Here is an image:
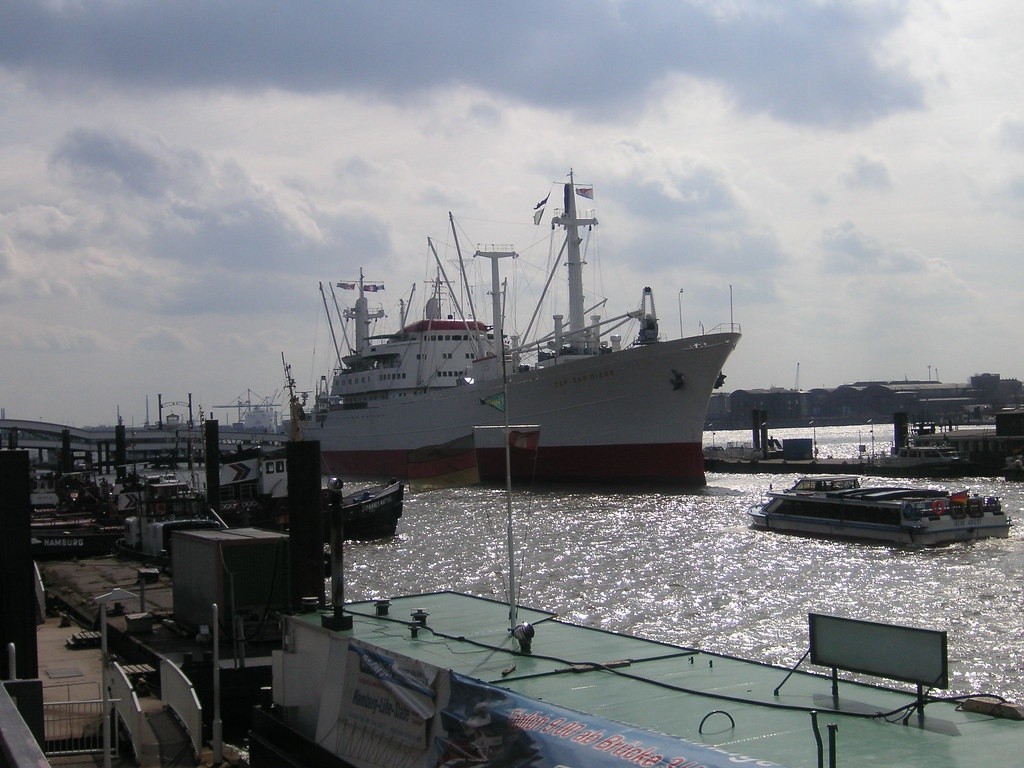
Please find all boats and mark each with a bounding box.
[276,165,743,487]
[751,473,1013,545]
[323,478,415,541]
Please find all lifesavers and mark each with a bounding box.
[904,503,914,517]
[966,498,986,519]
[948,500,967,520]
[932,500,945,516]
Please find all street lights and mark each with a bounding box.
[866,419,874,463]
[761,422,770,461]
[707,422,716,449]
[809,420,819,459]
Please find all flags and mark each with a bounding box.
[576,188,593,199]
[535,197,547,209]
[364,285,384,292]
[951,490,967,503]
[346,284,355,290]
[337,283,347,289]
[533,210,544,225]
